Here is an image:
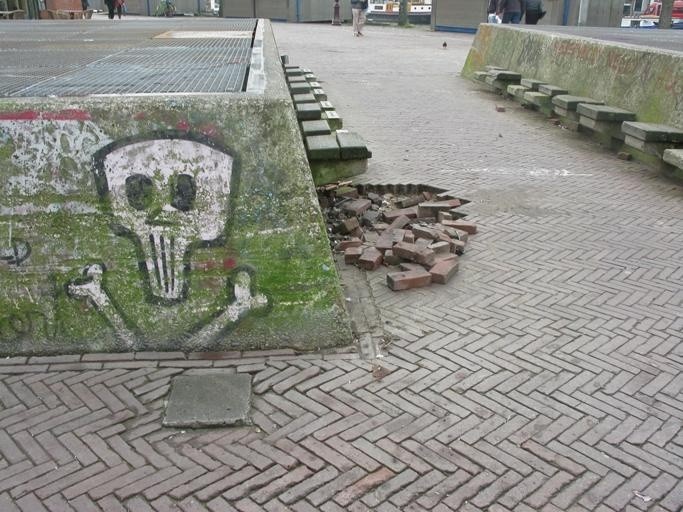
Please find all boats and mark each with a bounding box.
[364,0,432,25]
[619,4,683,29]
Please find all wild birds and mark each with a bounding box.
[443,41,447,48]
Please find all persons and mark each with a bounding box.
[104,0,114,19]
[486,0,499,24]
[522,0,547,25]
[495,0,523,25]
[349,0,369,38]
[112,0,124,20]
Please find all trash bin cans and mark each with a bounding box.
[332,0,341,26]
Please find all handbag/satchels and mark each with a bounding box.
[538,0,546,18]
